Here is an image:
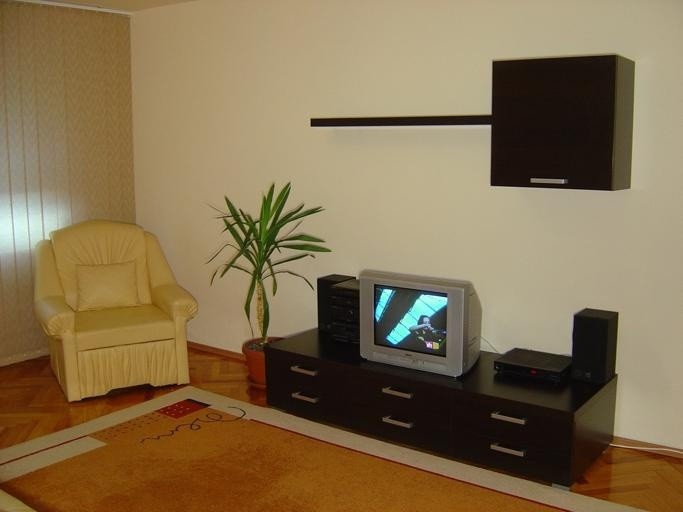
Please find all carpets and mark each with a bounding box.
[0,385,645,512]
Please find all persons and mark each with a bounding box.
[408,314,446,354]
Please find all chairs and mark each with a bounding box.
[33,220,200,403]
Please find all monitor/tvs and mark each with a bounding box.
[360,269,481,378]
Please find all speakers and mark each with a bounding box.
[574,308,618,381]
[317,275,354,336]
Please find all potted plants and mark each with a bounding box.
[201,181,332,385]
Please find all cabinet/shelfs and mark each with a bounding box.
[491,54,636,191]
[265,327,618,487]
